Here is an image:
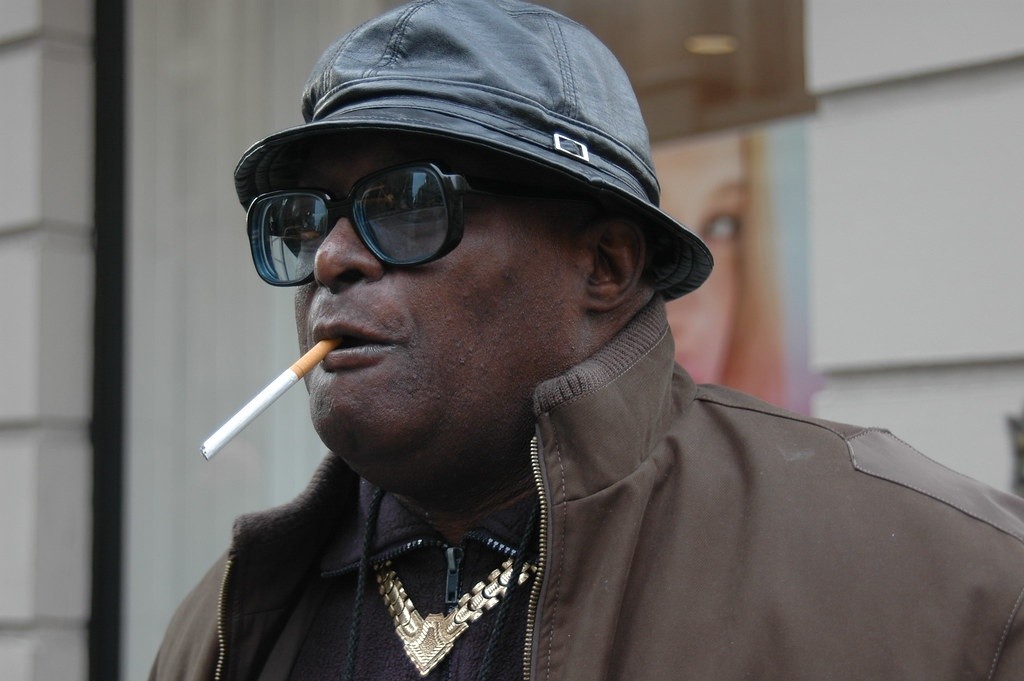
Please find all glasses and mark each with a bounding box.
[246,159,560,287]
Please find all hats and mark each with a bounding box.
[234,0,715,300]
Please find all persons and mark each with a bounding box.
[652,126,780,396]
[146,0,1024,680]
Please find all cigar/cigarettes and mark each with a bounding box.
[201,338,338,460]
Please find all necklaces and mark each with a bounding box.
[377,560,540,677]
